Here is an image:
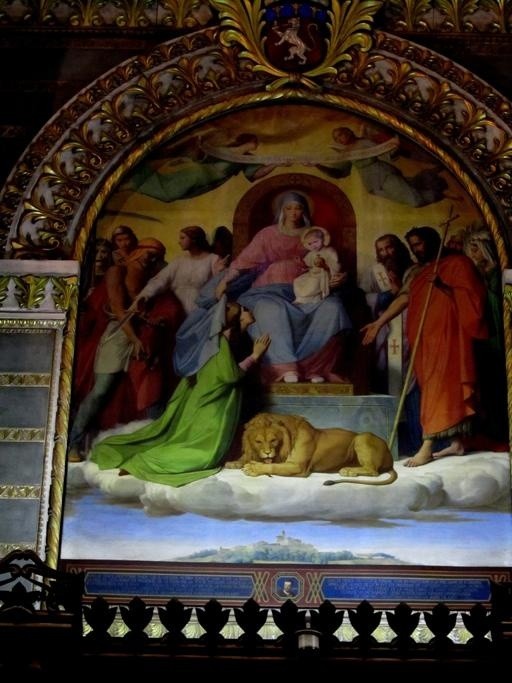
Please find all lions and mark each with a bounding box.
[223,411,397,487]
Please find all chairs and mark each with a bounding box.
[0,548,87,681]
[228,171,375,395]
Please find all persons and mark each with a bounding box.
[441,224,498,273]
[357,220,489,469]
[357,233,422,458]
[217,192,358,387]
[291,225,342,307]
